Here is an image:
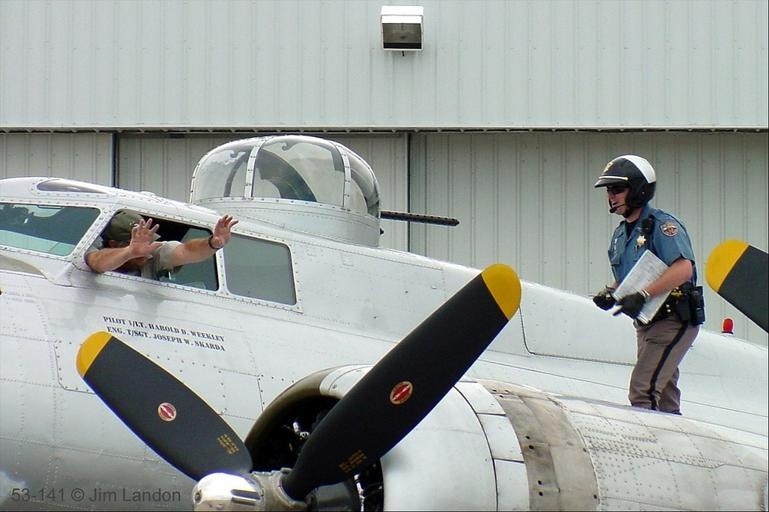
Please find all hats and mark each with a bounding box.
[101,210,160,245]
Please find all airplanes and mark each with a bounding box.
[0,133,769,512]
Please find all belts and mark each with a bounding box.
[633,300,673,329]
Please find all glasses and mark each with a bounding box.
[604,182,630,196]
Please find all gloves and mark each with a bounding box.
[611,291,651,319]
[593,286,617,311]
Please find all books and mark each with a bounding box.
[612,249,672,325]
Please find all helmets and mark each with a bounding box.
[594,153,656,213]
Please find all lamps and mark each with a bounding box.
[379,5,424,56]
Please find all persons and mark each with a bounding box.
[82,209,239,281]
[592,154,704,418]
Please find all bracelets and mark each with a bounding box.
[208,235,221,251]
[641,289,652,303]
